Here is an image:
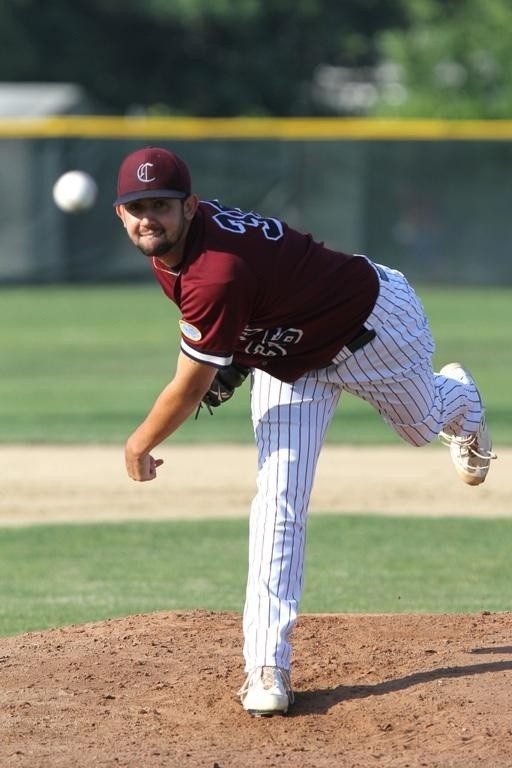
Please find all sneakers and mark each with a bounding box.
[438,361,498,484]
[236,666,295,716]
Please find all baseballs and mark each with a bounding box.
[50,169,98,213]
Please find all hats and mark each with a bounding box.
[112,146,191,207]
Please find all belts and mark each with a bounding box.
[321,262,390,368]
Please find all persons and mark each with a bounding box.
[104,142,503,717]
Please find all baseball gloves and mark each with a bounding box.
[194,360,250,420]
